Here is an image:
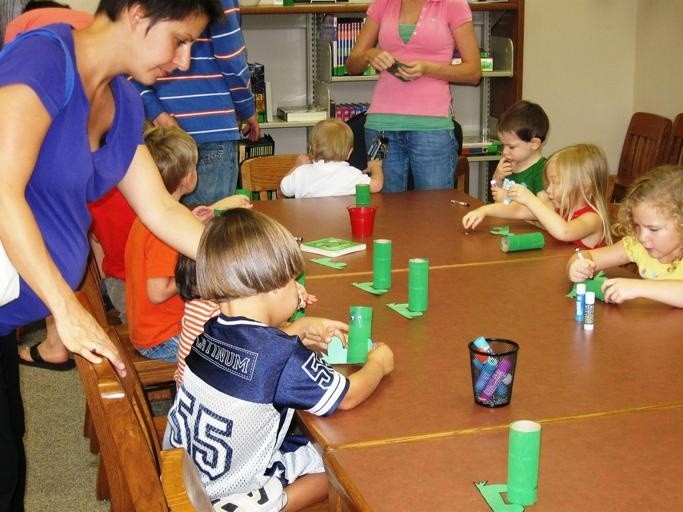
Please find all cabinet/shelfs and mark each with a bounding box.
[239,0,525,202]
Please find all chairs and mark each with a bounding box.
[602,111,674,209]
[239,154,313,199]
[67,257,197,512]
[660,113,682,168]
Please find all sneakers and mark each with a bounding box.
[211,476,287,512]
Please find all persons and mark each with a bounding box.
[175,209,395,511]
[0,0,318,511]
[88,125,138,320]
[174,250,326,395]
[121,1,258,206]
[462,142,611,252]
[487,101,550,206]
[566,166,683,310]
[345,1,482,194]
[277,118,384,200]
[124,124,254,361]
[4,1,94,372]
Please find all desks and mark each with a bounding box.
[323,403,683,512]
[179,187,585,279]
[289,254,683,450]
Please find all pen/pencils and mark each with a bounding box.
[451,199,471,207]
[576,249,595,282]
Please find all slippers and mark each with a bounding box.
[17,341,74,370]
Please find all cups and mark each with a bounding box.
[346,204,377,240]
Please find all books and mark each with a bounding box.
[276,102,326,121]
[324,15,377,76]
[329,100,371,121]
